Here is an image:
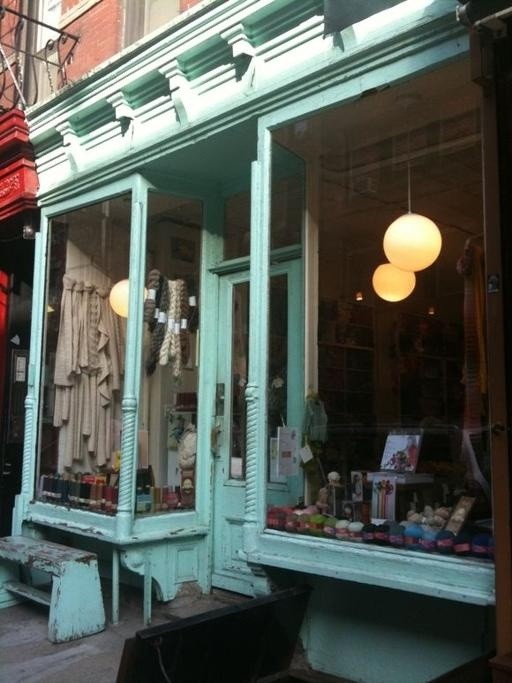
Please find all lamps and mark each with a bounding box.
[370,95,444,302]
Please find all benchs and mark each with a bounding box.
[0,533,109,646]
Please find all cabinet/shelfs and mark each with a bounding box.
[313,299,463,440]
